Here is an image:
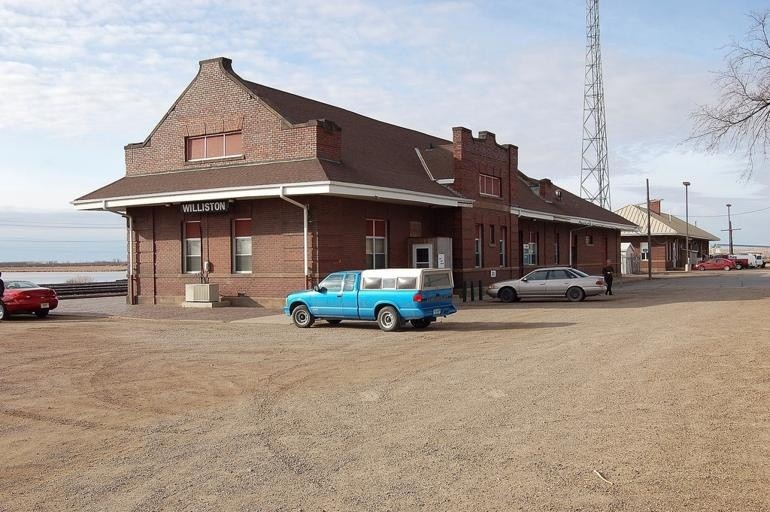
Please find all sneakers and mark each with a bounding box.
[605,292,614,296]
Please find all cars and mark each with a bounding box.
[1,280,59,323]
[485,265,608,303]
[694,252,767,271]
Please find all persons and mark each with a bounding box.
[601,258,616,296]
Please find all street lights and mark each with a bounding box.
[726,203,735,254]
[683,181,692,273]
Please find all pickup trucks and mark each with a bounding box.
[284,265,459,331]
